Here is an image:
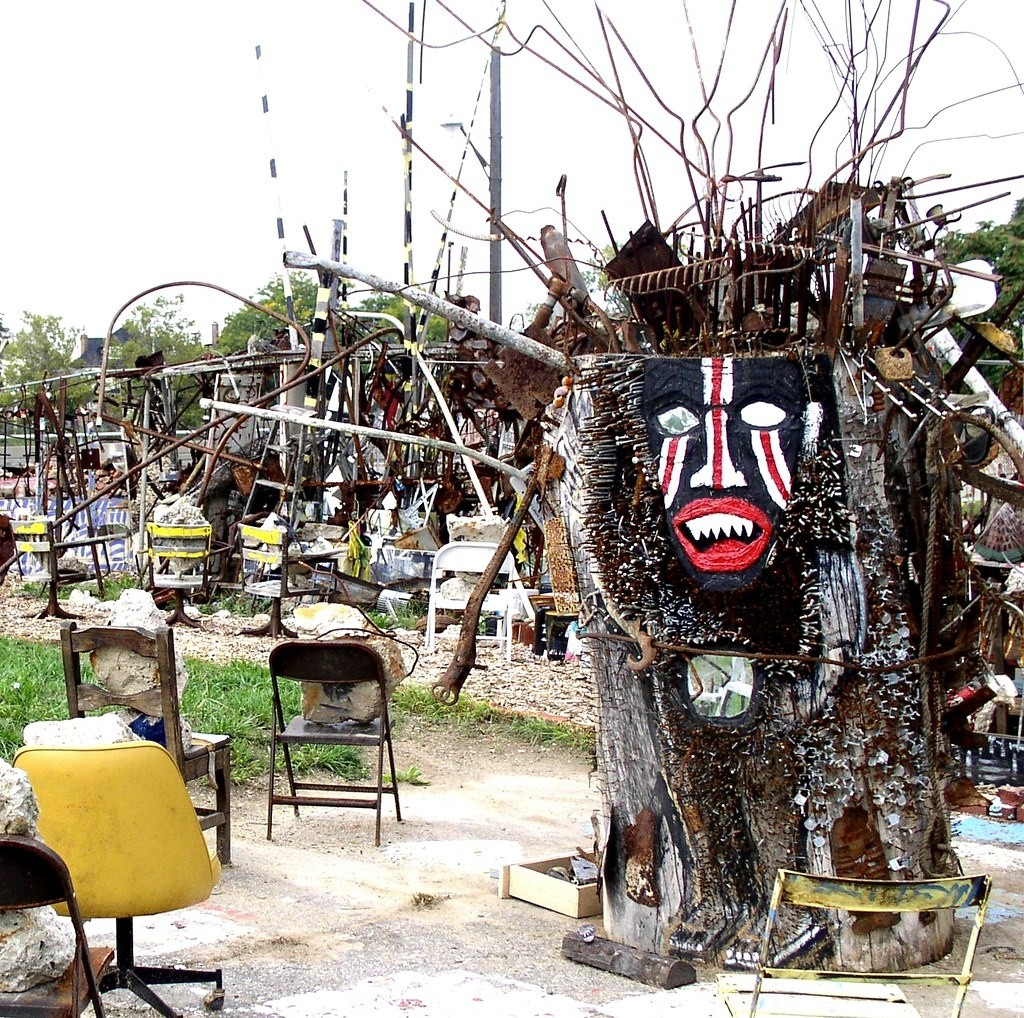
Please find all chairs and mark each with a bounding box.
[714,868,995,1017]
[0,833,114,1018]
[236,524,337,641]
[59,621,231,864]
[266,640,402,846]
[423,541,515,662]
[10,740,225,1018]
[145,523,233,629]
[8,520,128,621]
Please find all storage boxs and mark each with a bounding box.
[499,848,603,919]
